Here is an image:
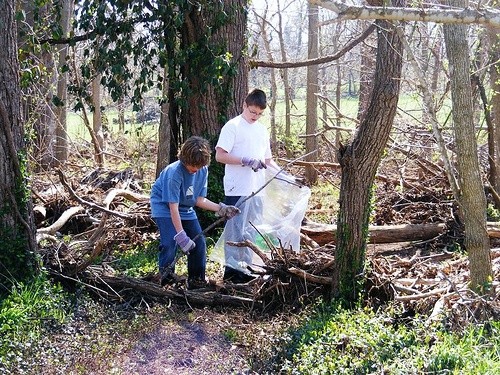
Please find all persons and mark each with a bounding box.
[215,89,309,284]
[150,136,242,290]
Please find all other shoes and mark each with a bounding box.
[187,280,210,289]
[161,276,182,288]
[222,266,261,283]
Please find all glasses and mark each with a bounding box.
[247,107,265,117]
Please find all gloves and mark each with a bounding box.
[215,202,242,220]
[174,230,196,255]
[295,176,311,187]
[241,156,267,173]
